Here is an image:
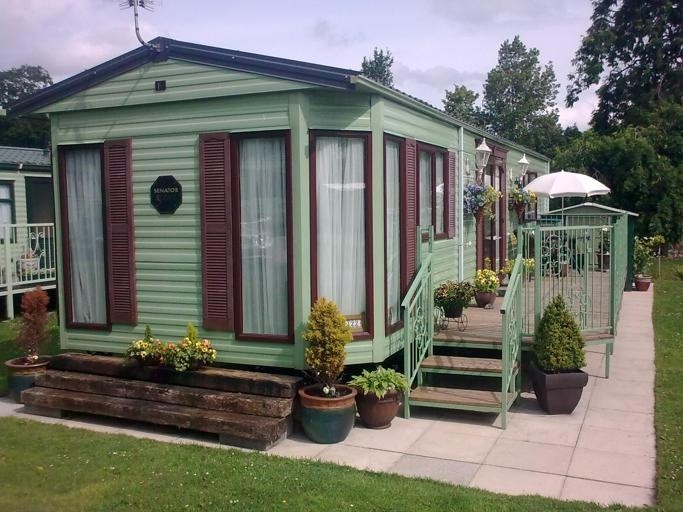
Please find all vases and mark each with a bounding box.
[476,292,494,307]
[434,303,468,333]
[515,204,526,221]
[474,205,482,225]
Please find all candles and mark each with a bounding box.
[295,297,358,444]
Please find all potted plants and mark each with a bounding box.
[629,238,654,291]
[593,218,611,268]
[3,286,51,404]
[347,365,408,430]
[527,293,588,414]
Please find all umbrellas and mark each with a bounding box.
[524,168,612,277]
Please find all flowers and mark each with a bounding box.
[510,187,536,205]
[500,256,534,276]
[434,276,476,306]
[464,181,500,213]
[125,324,218,372]
[473,268,498,292]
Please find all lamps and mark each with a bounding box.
[509,152,528,183]
[464,137,492,177]
